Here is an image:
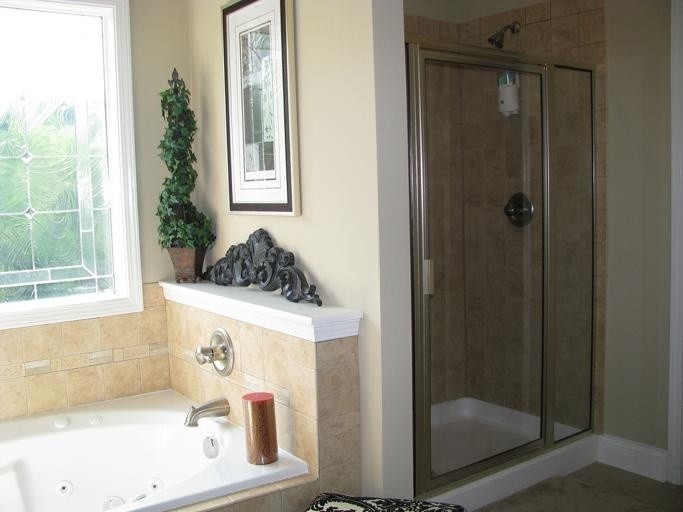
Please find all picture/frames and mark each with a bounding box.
[218,0,303,218]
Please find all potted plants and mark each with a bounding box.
[157,66,215,284]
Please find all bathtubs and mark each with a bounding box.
[0,387,308,512]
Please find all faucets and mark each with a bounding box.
[183,400,231,427]
